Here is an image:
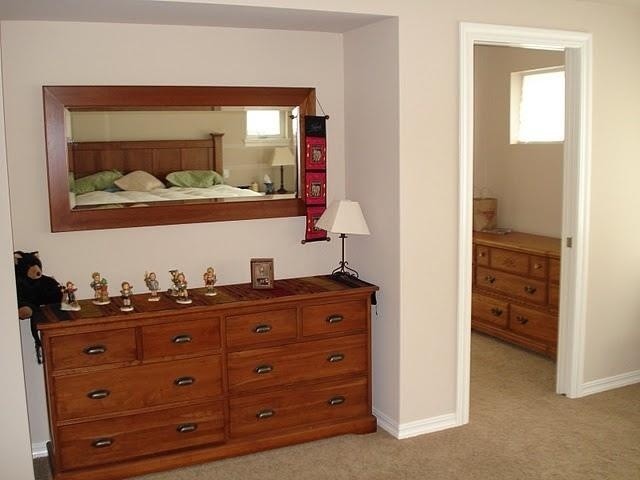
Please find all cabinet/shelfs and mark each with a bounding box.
[473,228,562,361]
[35,274,379,480]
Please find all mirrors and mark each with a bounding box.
[43,85,318,233]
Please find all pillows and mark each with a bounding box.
[69,169,224,197]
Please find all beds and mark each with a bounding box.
[67,133,263,207]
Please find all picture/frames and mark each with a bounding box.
[250,258,274,290]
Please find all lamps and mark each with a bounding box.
[314,199,371,281]
[268,146,296,193]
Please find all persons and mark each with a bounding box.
[149,273,159,297]
[64,282,77,304]
[205,267,216,294]
[120,281,133,308]
[176,273,188,301]
[169,270,179,291]
[90,271,102,301]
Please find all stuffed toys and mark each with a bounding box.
[15,251,63,363]
